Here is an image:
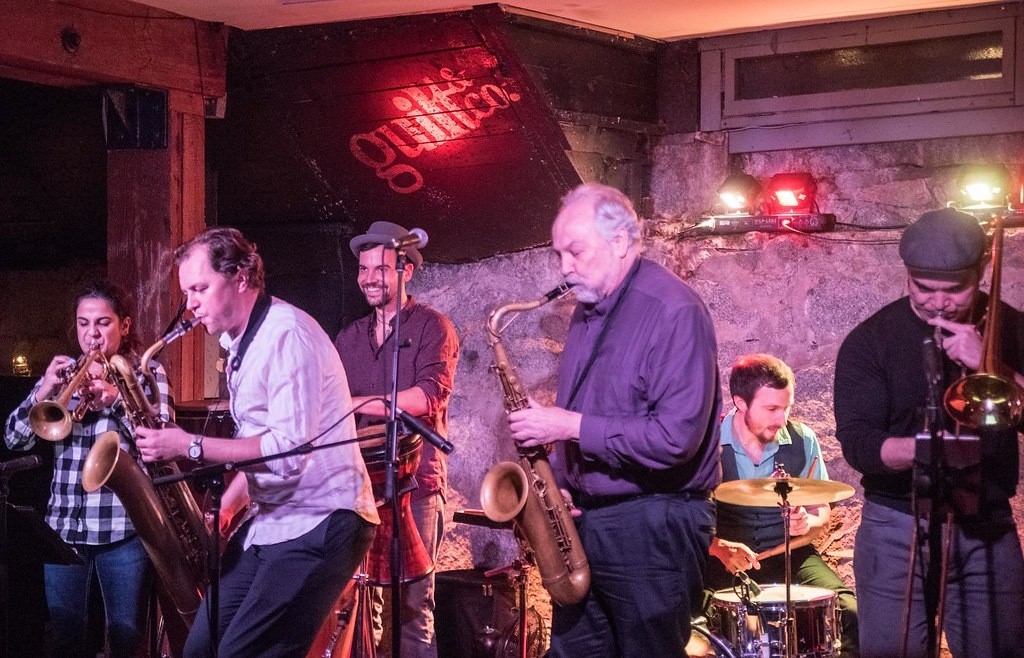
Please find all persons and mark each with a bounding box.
[503,184,722,658]
[329,223,459,657]
[834,209,1024,658]
[3,282,174,654]
[698,352,874,641]
[135,227,382,658]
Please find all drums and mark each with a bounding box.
[174,398,235,443]
[354,423,436,588]
[705,581,844,658]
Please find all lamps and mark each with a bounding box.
[954,161,1024,227]
[11,352,33,377]
[713,171,833,232]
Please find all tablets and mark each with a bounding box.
[911,435,982,515]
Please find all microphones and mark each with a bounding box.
[0,454,41,476]
[382,398,454,456]
[920,332,944,389]
[384,228,429,250]
[739,571,762,597]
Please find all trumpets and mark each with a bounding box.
[29,342,106,443]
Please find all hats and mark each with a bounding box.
[899,209,984,273]
[345,222,423,267]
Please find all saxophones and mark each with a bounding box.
[78,315,215,638]
[478,281,590,605]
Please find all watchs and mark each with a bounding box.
[187,434,206,464]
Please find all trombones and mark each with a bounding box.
[898,203,1024,658]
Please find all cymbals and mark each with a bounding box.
[711,474,856,510]
